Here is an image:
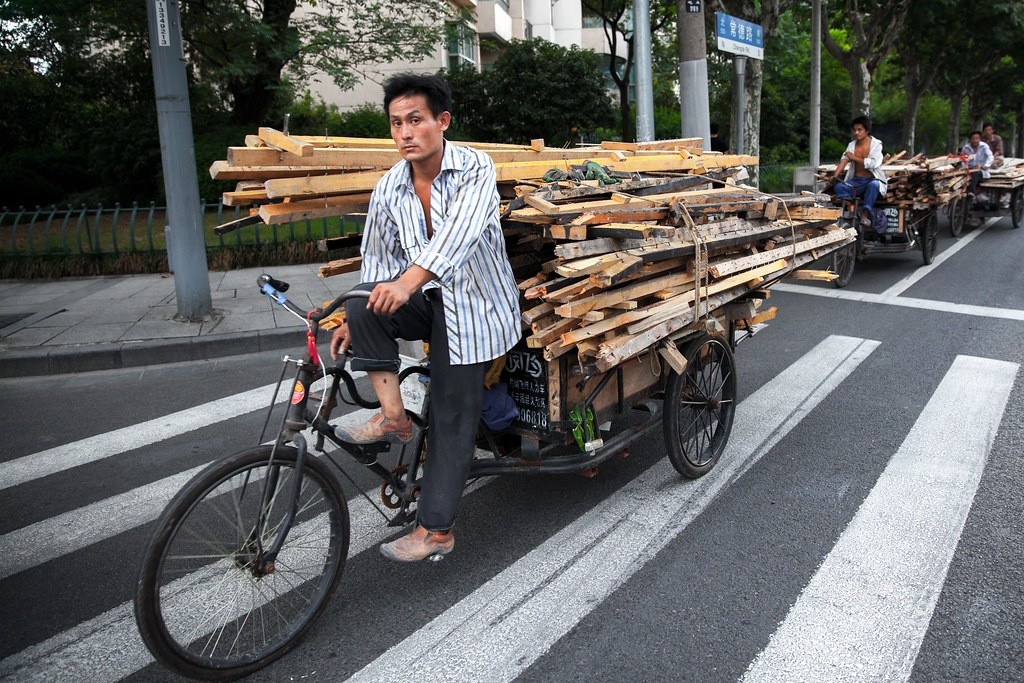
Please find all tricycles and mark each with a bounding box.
[947,168,1024,238]
[132,270,772,683]
[830,172,958,289]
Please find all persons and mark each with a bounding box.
[710,123,729,189]
[829,115,888,226]
[330,72,521,562]
[960,122,1004,198]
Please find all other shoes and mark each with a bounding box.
[966,192,974,204]
[860,215,871,226]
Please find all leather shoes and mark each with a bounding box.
[380,524,455,562]
[334,413,414,444]
